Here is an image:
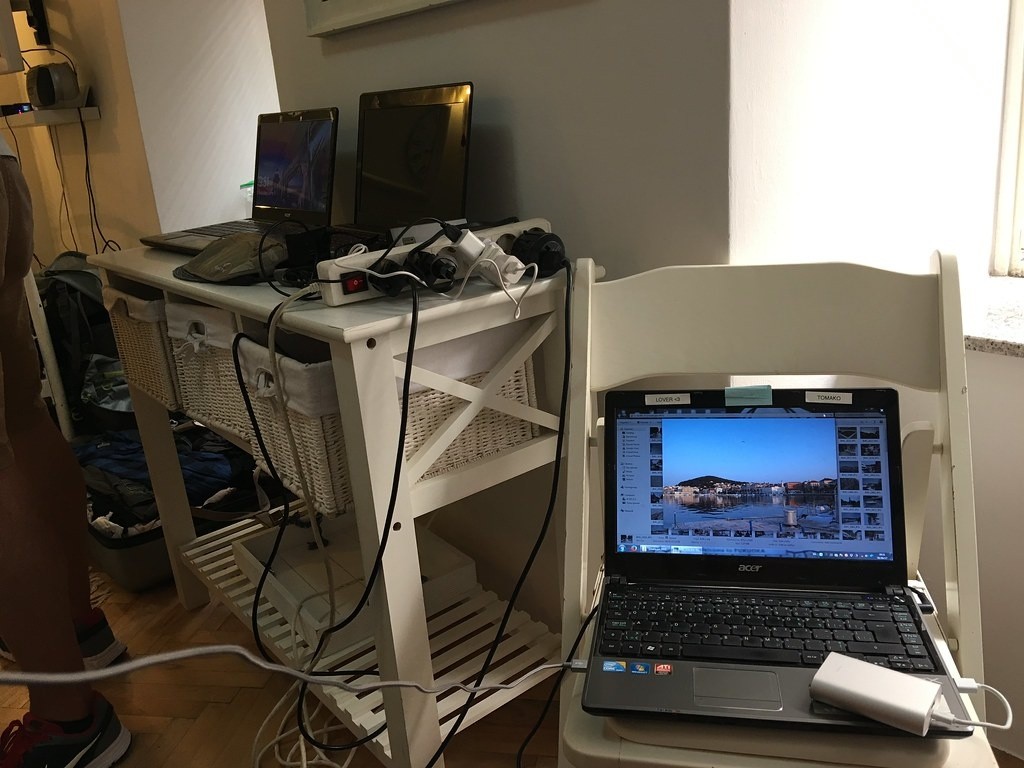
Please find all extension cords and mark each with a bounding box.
[317,217,550,307]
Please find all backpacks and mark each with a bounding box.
[31,251,138,437]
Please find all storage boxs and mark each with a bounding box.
[231,514,478,659]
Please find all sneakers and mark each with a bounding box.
[0,688,131,768]
[0,606,128,669]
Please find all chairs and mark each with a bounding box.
[557,249,999,768]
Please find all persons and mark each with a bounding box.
[0,139,132,768]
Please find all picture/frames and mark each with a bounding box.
[303,0,462,38]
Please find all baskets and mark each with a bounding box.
[101,285,183,412]
[232,334,541,520]
[164,303,250,442]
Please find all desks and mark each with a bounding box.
[86,245,607,768]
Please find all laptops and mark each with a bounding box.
[578,387,974,740]
[142,81,474,290]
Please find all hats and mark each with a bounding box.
[172,231,289,283]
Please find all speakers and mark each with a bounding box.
[37,61,85,110]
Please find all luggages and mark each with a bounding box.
[87,501,233,594]
